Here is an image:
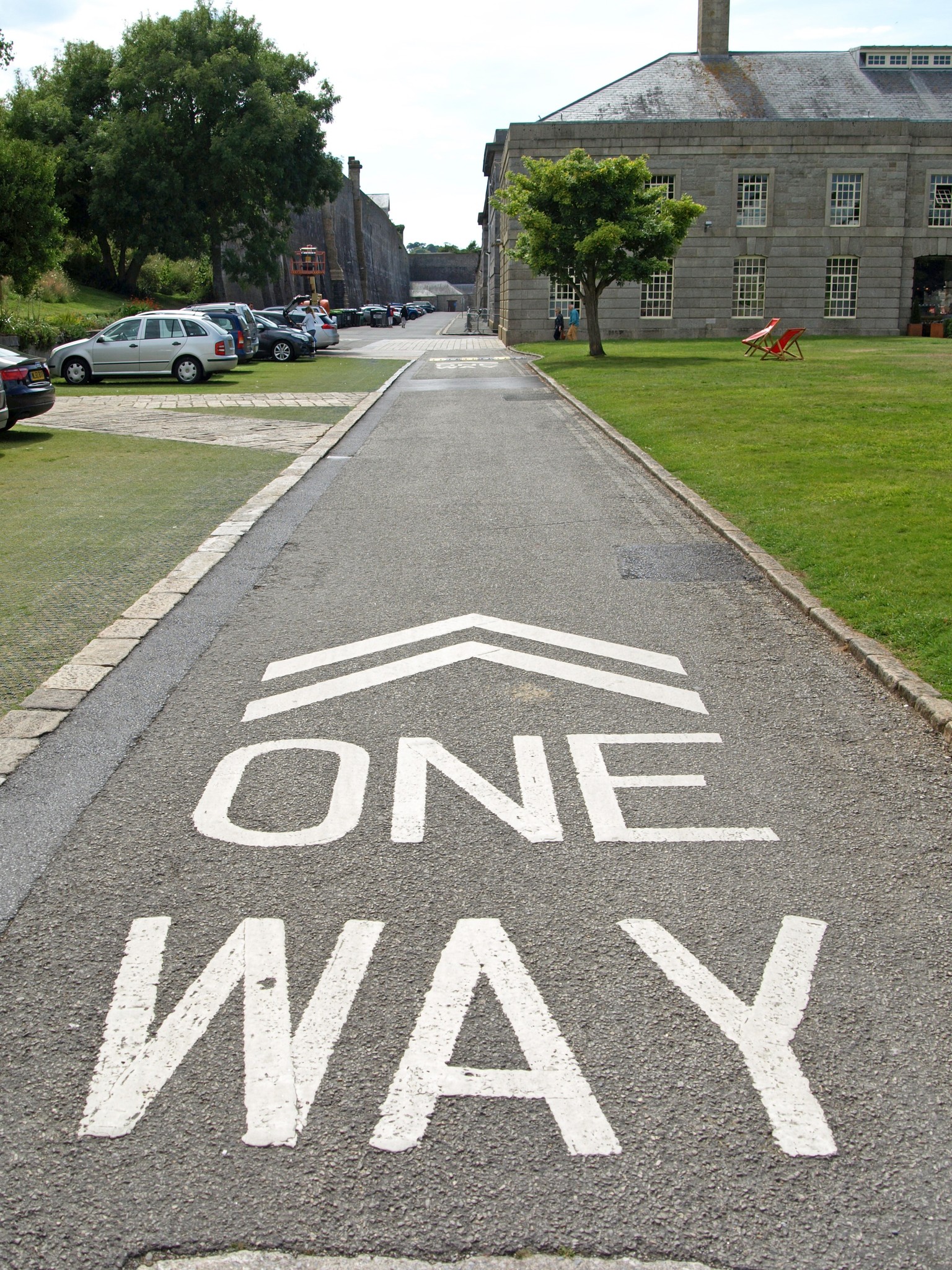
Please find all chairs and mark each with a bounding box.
[742,317,807,361]
[185,325,202,335]
[145,321,157,338]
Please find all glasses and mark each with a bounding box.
[250,306,254,307]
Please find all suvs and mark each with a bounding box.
[262,304,330,318]
[181,302,260,355]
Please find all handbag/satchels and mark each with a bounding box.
[390,308,394,316]
[562,329,566,339]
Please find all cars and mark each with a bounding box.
[190,309,254,364]
[0,344,57,432]
[218,314,317,362]
[271,310,339,349]
[358,306,402,326]
[251,294,313,333]
[103,310,213,352]
[48,314,238,385]
[362,303,434,321]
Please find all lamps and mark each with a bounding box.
[485,251,492,255]
[704,221,713,226]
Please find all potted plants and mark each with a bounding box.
[926,306,944,337]
[906,295,923,337]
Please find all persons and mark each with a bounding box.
[386,304,393,328]
[248,303,256,311]
[296,307,317,359]
[553,307,564,341]
[399,306,408,328]
[566,305,580,341]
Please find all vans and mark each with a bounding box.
[413,301,436,311]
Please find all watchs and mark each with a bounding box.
[559,327,560,328]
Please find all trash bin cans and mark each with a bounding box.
[356,312,364,326]
[370,308,382,327]
[382,309,388,327]
[329,308,357,329]
